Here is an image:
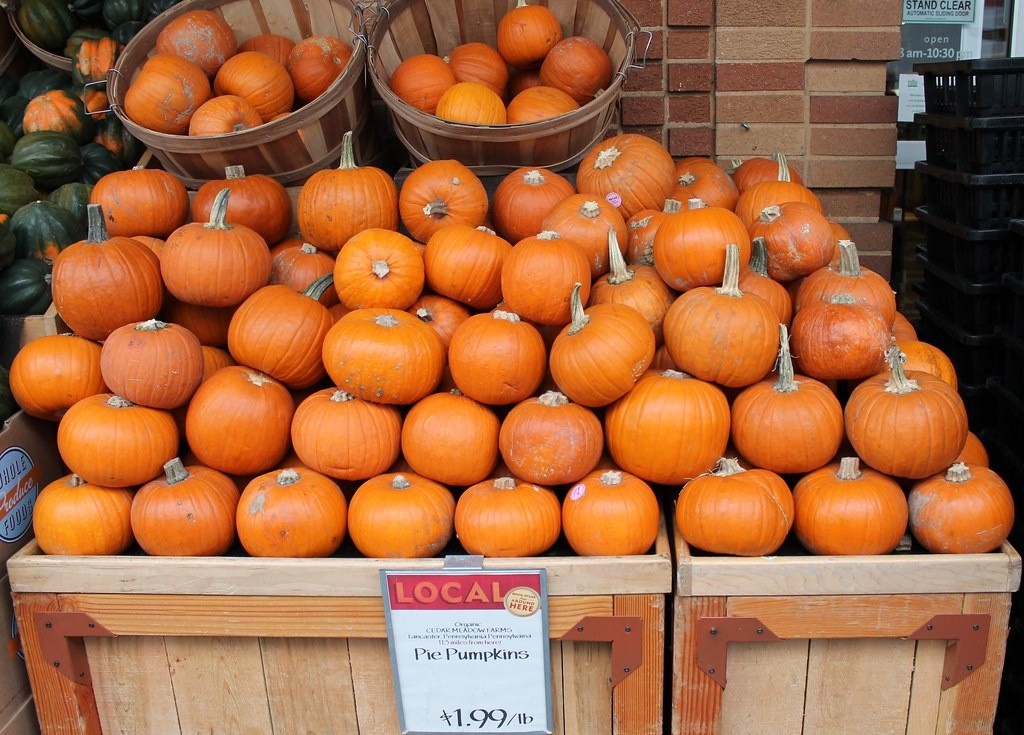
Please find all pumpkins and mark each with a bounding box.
[0,0,1015,557]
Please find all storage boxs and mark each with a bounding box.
[0,410,80,735]
[911,56,1024,735]
